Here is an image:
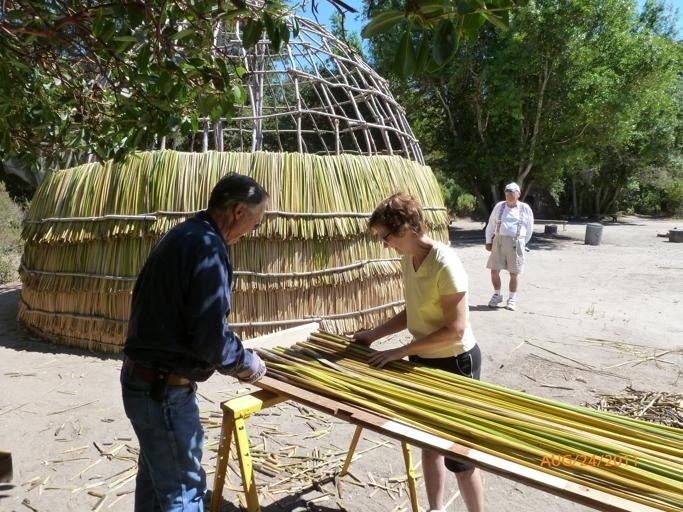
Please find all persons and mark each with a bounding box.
[352,192,487,512]
[121,170,270,512]
[484,182,534,311]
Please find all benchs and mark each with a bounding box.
[533,217,568,231]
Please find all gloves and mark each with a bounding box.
[236,349,267,384]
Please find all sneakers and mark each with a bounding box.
[488,294,503,308]
[506,299,516,311]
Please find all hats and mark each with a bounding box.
[504,182,520,192]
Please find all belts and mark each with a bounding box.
[125,358,190,385]
